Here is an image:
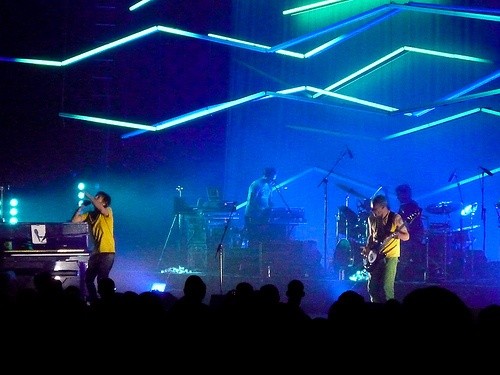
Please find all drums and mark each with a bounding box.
[332,211,472,283]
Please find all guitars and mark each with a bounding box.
[363,209,421,272]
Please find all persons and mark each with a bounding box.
[361,195,410,303]
[0,271,500,375]
[72,190,116,299]
[395,184,424,251]
[244,167,277,242]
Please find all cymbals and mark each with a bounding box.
[426,200,463,214]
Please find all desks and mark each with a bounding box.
[3,250,93,299]
[256,222,308,241]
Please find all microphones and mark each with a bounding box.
[479,165,493,176]
[347,146,354,158]
[224,202,237,205]
[448,172,456,183]
